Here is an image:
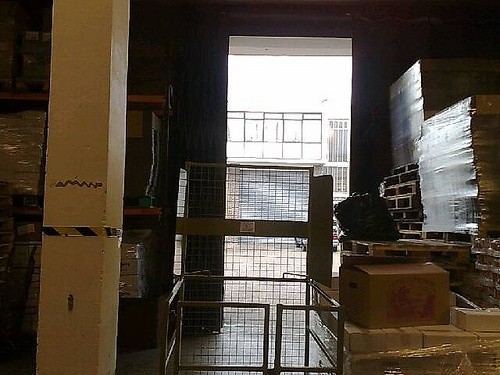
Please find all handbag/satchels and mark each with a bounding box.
[335,191,401,241]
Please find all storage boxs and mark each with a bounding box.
[308,271,500,375]
[338,255,449,328]
[4,220,42,339]
[119,229,158,299]
